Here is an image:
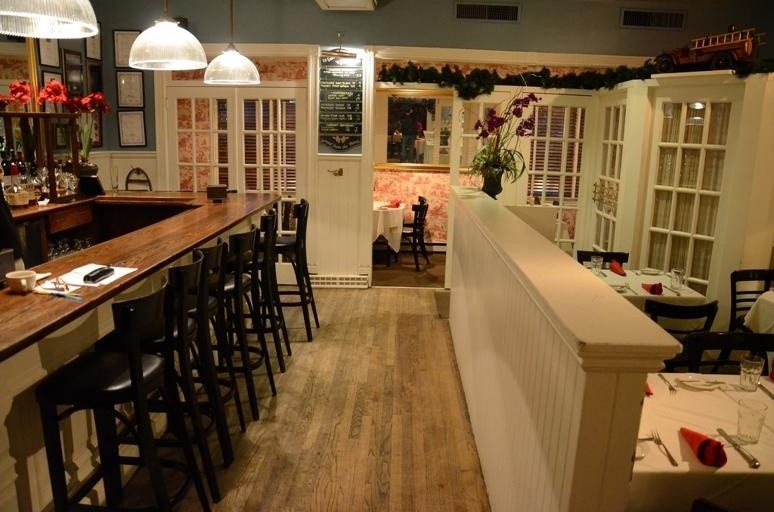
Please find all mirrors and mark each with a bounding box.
[372,87,456,174]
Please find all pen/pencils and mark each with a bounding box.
[32,289,83,302]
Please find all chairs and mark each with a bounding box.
[169,236,246,436]
[33,275,212,512]
[574,247,631,273]
[640,297,719,374]
[111,249,236,505]
[681,330,774,376]
[188,224,277,421]
[257,197,320,343]
[708,268,772,375]
[388,202,432,272]
[245,205,292,373]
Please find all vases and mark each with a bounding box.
[62,156,101,178]
[478,162,503,201]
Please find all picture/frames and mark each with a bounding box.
[35,35,62,69]
[112,28,142,70]
[116,110,148,149]
[60,47,87,97]
[37,77,67,116]
[115,71,145,110]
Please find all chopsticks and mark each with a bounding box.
[663,285,680,296]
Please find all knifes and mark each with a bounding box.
[716,428,761,469]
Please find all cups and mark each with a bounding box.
[739,354,766,393]
[591,256,603,277]
[671,267,684,290]
[5,270,37,293]
[737,398,768,443]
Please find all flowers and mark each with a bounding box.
[0,79,35,113]
[464,83,546,185]
[61,89,111,164]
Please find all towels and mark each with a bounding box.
[610,259,628,276]
[680,426,728,469]
[385,199,404,209]
[642,282,663,295]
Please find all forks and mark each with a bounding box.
[650,430,679,467]
[625,283,639,296]
[658,373,677,394]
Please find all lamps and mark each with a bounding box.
[203,0,265,89]
[0,0,100,42]
[127,0,206,76]
[319,29,359,61]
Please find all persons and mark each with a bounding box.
[400,107,416,162]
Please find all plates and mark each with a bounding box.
[641,268,661,274]
[677,372,714,390]
[613,285,626,293]
